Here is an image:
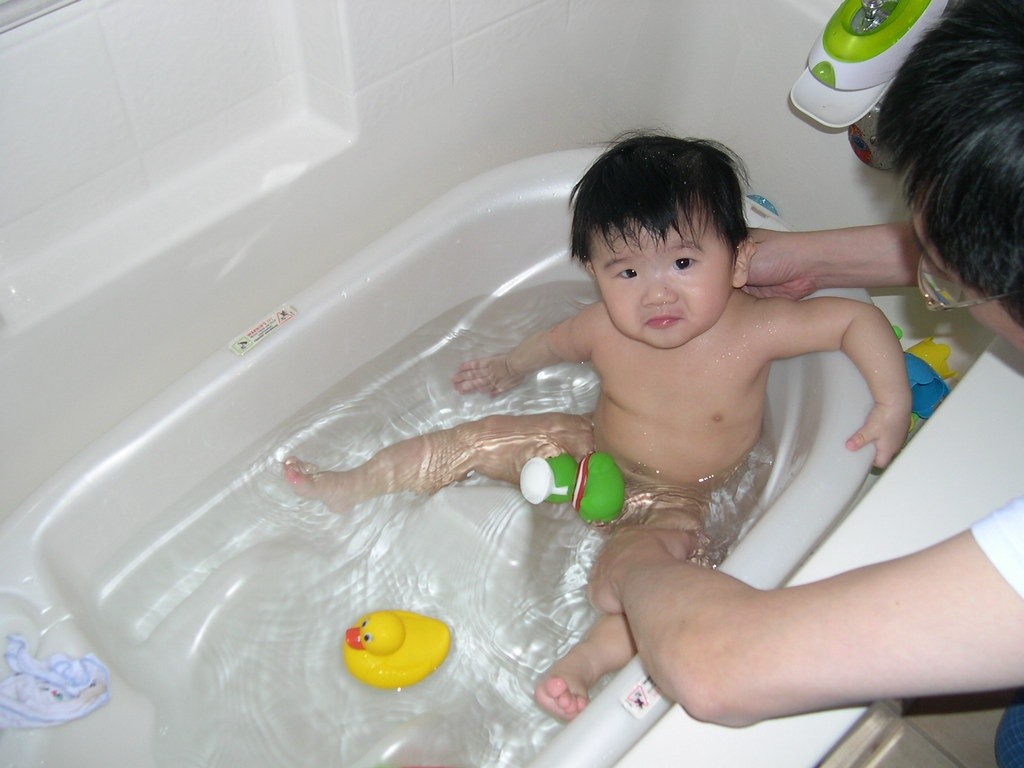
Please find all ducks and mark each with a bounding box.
[342,609,454,691]
[519,451,626,524]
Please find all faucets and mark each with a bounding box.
[789,1,948,130]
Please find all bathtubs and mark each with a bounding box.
[1,147,876,768]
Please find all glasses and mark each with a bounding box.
[917,250,1024,313]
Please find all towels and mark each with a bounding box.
[1,635,112,728]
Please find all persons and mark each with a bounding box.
[283,129,911,724]
[588,0,1024,768]
